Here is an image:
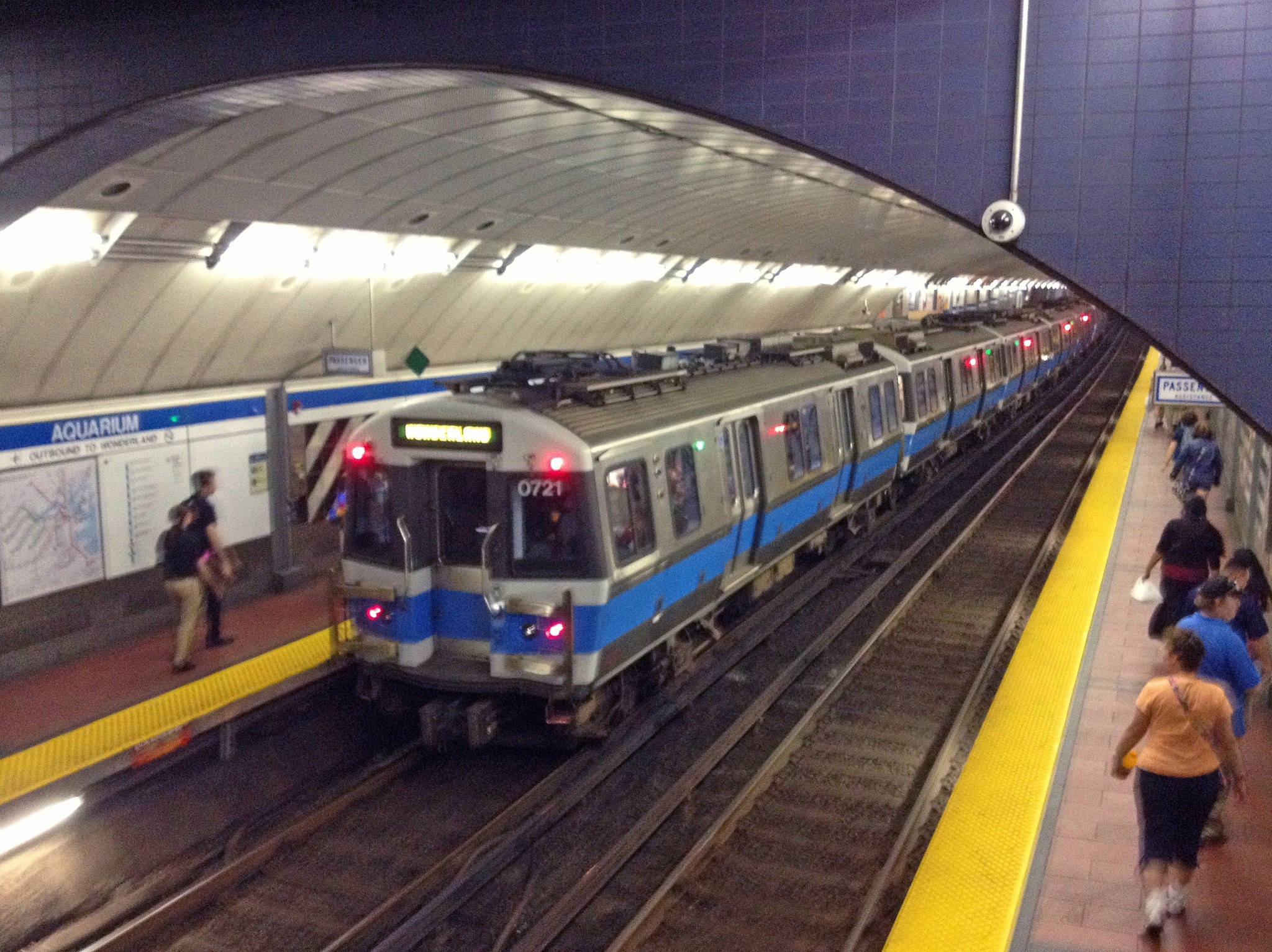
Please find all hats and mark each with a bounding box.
[1198,576,1242,596]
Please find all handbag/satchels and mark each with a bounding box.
[1171,477,1190,497]
[1219,769,1228,787]
[1130,576,1161,604]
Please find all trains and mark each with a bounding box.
[327,296,1109,743]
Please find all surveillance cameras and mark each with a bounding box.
[982,201,1026,243]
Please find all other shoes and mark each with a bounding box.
[1200,811,1221,841]
[172,662,195,674]
[1167,891,1186,914]
[1139,899,1164,934]
[1155,422,1163,428]
[206,637,233,648]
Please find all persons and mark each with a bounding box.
[326,471,348,554]
[1110,626,1248,937]
[1139,494,1272,729]
[154,469,236,673]
[1178,575,1265,846]
[1148,358,1222,498]
